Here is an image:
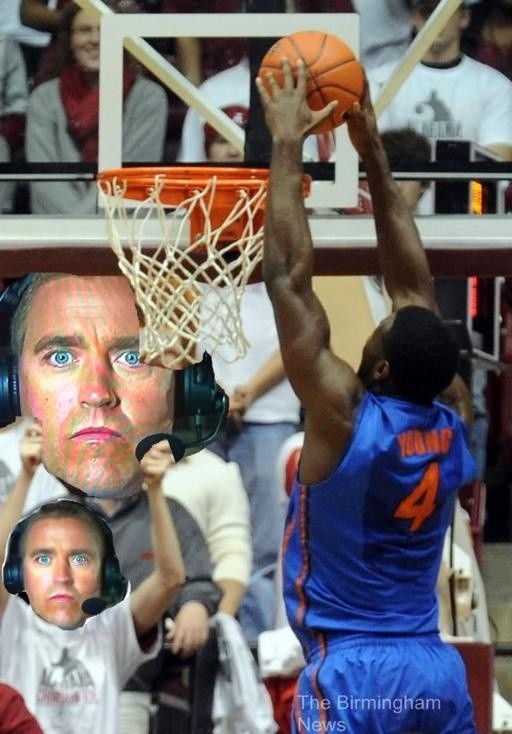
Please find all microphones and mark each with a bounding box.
[80,579,132,614]
[135,386,231,464]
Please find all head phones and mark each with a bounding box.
[1,269,222,431]
[2,499,123,601]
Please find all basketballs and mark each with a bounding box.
[259,31,365,132]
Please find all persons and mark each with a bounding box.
[50,486,224,734]
[1,417,189,734]
[0,0,512,226]
[183,274,306,659]
[241,54,489,734]
[10,272,176,501]
[145,444,256,678]
